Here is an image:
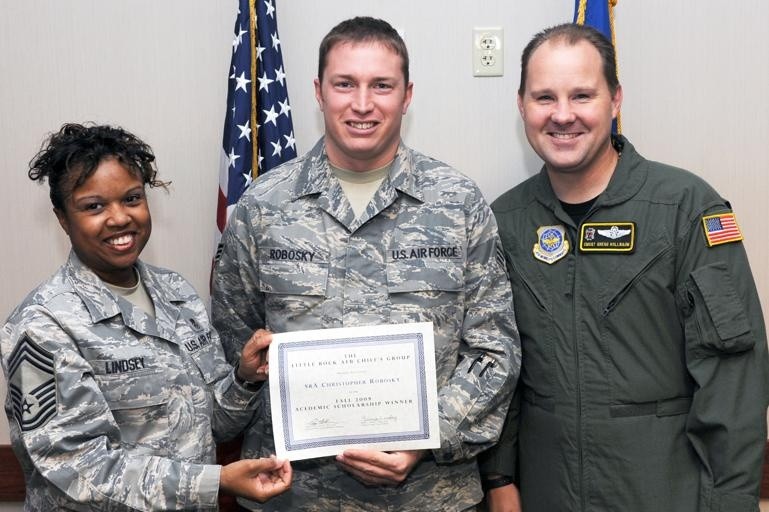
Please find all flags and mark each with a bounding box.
[217,0,297,235]
[572,0,621,136]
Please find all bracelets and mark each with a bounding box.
[483,476,513,489]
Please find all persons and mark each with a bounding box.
[0,121,293,512]
[477,23,769,512]
[209,16,523,512]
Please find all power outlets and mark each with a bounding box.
[473,26,504,77]
